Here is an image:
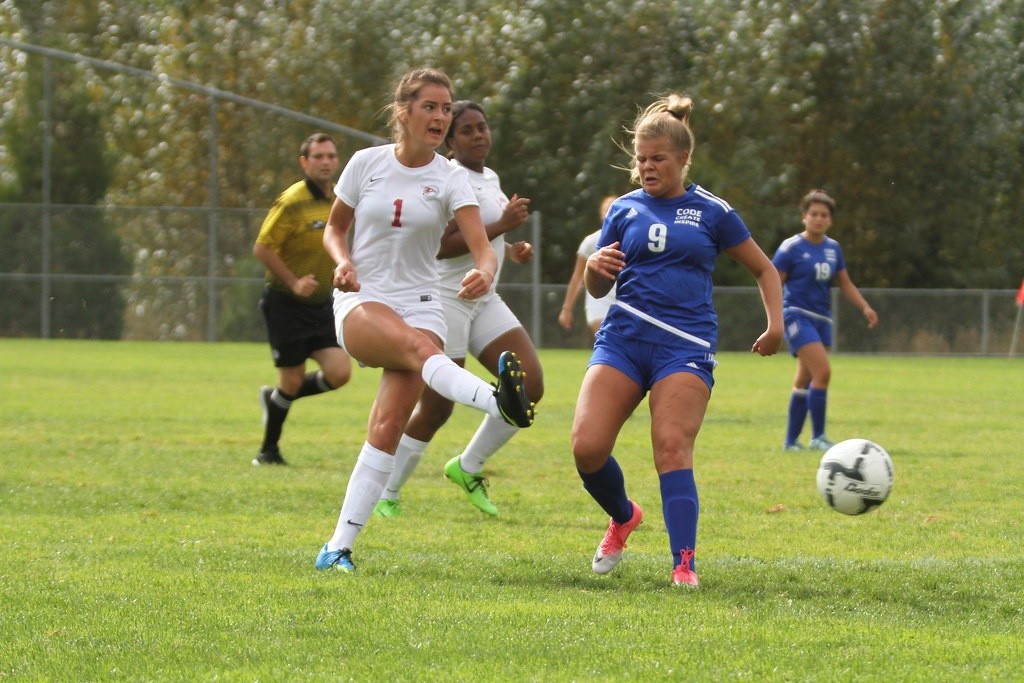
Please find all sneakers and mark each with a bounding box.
[808,433,832,449]
[593,500,642,575]
[252,448,286,467]
[783,441,804,450]
[375,499,402,520]
[672,546,700,589]
[490,351,539,429]
[315,544,356,574]
[442,455,499,516]
[260,385,273,424]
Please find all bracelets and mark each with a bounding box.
[561,305,572,312]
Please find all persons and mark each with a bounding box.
[557,195,618,338]
[372,100,545,518]
[573,91,785,590]
[772,193,881,452]
[250,132,350,471]
[314,67,536,576]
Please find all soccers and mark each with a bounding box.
[815,438,893,516]
